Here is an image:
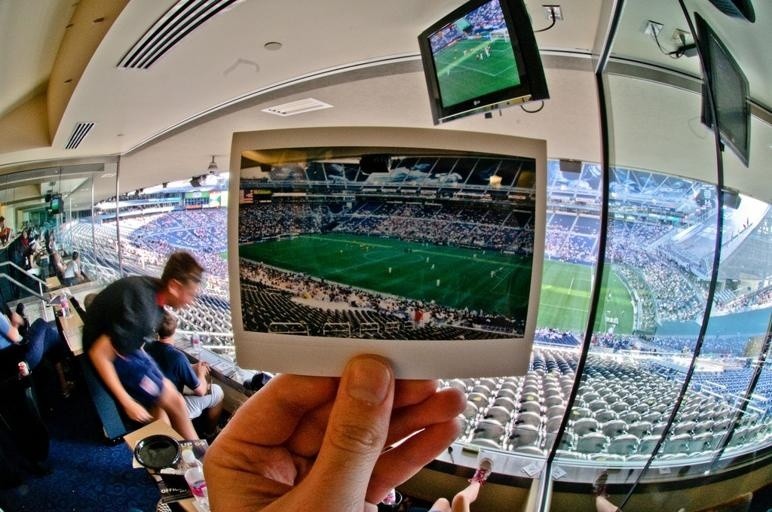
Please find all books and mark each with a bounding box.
[141,437,213,505]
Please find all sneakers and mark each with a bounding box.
[204,424,225,438]
[64,381,73,397]
[594,472,607,495]
[476,458,493,483]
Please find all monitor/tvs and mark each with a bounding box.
[692,9,754,171]
[417,0,551,126]
[50,198,65,214]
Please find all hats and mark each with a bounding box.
[243,373,271,391]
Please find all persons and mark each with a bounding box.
[143,313,225,436]
[202,355,467,511]
[0,178,772,381]
[427,455,494,511]
[590,471,623,512]
[81,245,203,443]
[427,0,509,62]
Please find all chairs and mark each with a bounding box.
[167,293,233,366]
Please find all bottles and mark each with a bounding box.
[182,449,211,512]
[191,331,201,355]
[60,292,71,318]
[381,487,397,506]
